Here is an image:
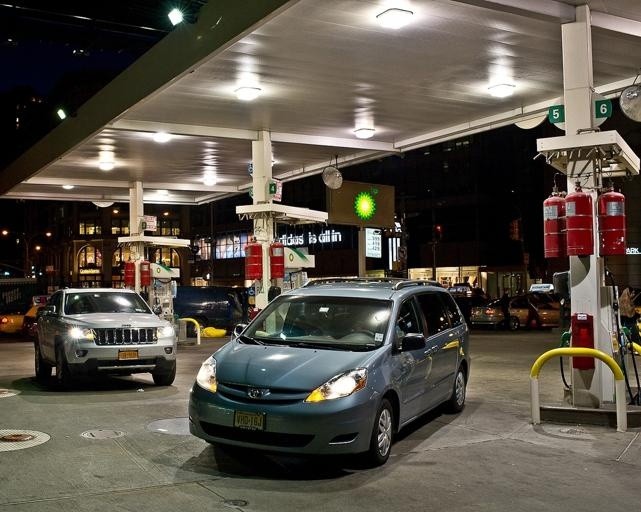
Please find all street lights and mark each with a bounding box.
[2,230,52,278]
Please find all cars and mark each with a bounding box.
[189,277,471,465]
[469,284,562,330]
[0,288,176,390]
[177,285,244,337]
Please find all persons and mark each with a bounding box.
[619,286,635,342]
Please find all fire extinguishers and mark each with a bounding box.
[125,255,150,286]
[543,180,626,258]
[245,237,284,280]
